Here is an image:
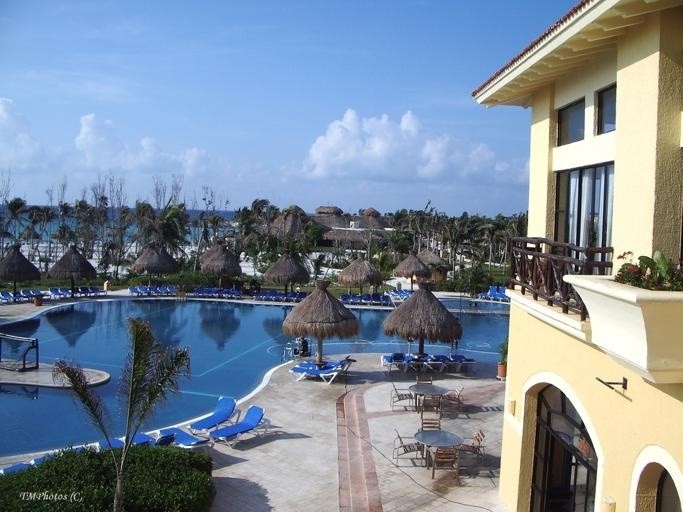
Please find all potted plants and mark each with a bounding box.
[494,334,508,378]
[561,248,683,372]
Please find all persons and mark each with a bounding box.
[296,339,310,357]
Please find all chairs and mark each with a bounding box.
[290,353,355,384]
[0,392,269,479]
[383,372,486,483]
[381,350,476,375]
[0,278,514,320]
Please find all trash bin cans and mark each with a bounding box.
[33,295,42,306]
